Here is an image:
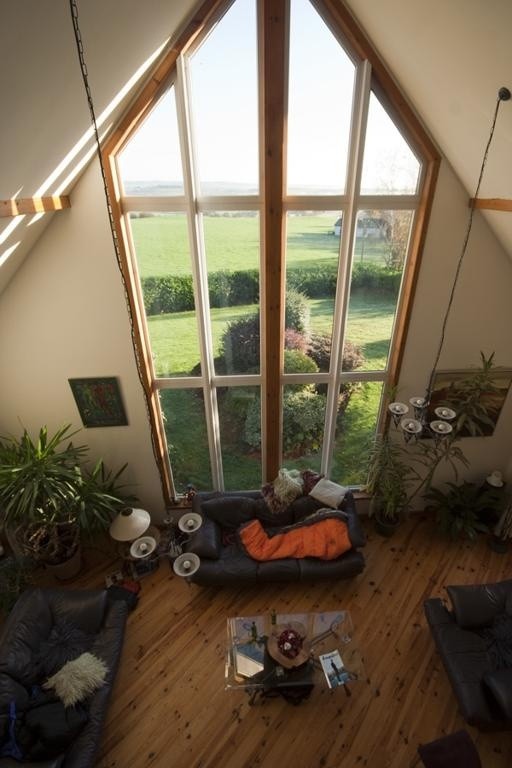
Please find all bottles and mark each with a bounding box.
[251,621,259,640]
[269,607,277,625]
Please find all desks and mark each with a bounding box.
[119,524,160,581]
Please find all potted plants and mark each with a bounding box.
[358,349,497,538]
[0,423,136,583]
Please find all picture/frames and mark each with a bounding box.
[68,377,128,428]
[414,367,512,440]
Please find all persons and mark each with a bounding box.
[330,658,341,682]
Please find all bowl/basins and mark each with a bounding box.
[265,621,311,671]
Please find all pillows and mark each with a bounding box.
[415,726,485,767]
[305,476,349,511]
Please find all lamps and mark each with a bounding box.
[68,0,205,585]
[384,83,510,448]
[107,505,154,551]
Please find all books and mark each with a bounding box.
[318,649,351,688]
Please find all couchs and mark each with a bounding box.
[185,487,368,592]
[1,583,131,768]
[423,576,511,732]
